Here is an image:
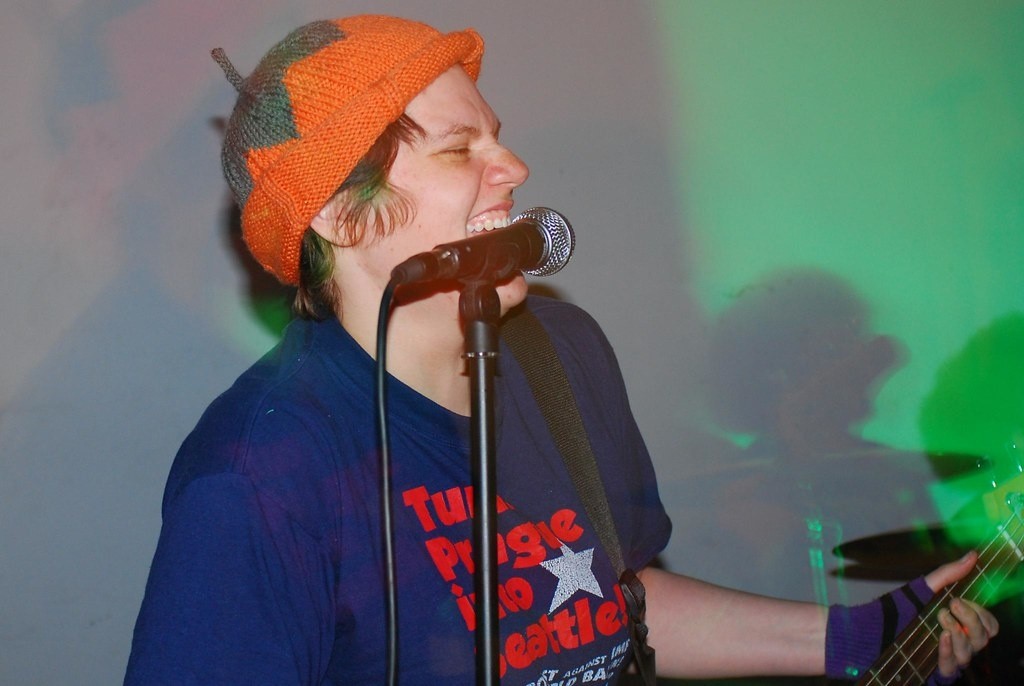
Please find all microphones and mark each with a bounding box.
[390,206,576,288]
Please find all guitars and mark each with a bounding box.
[851,438,1023,686]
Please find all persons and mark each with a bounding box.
[124,13,999,686]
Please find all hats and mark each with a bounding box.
[212,14,486,287]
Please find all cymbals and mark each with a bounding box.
[830,523,971,575]
